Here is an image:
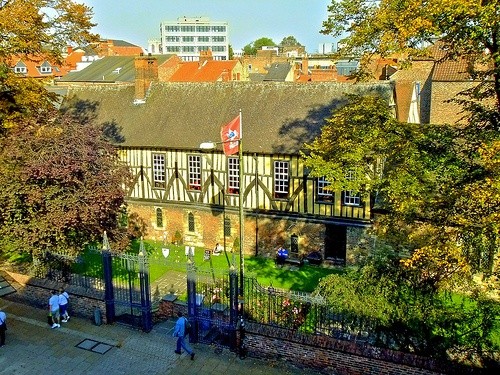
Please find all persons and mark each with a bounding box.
[49,286,71,328]
[171,311,195,359]
[0,309,7,348]
[278,245,289,267]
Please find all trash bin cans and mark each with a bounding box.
[93,309,102,325]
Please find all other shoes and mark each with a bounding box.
[57,323,61,328]
[67,316,70,321]
[175,350,181,354]
[52,323,57,328]
[62,320,68,323]
[190,352,195,360]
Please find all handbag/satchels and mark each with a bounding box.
[2,322,8,331]
[183,317,190,331]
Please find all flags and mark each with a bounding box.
[221,114,240,155]
